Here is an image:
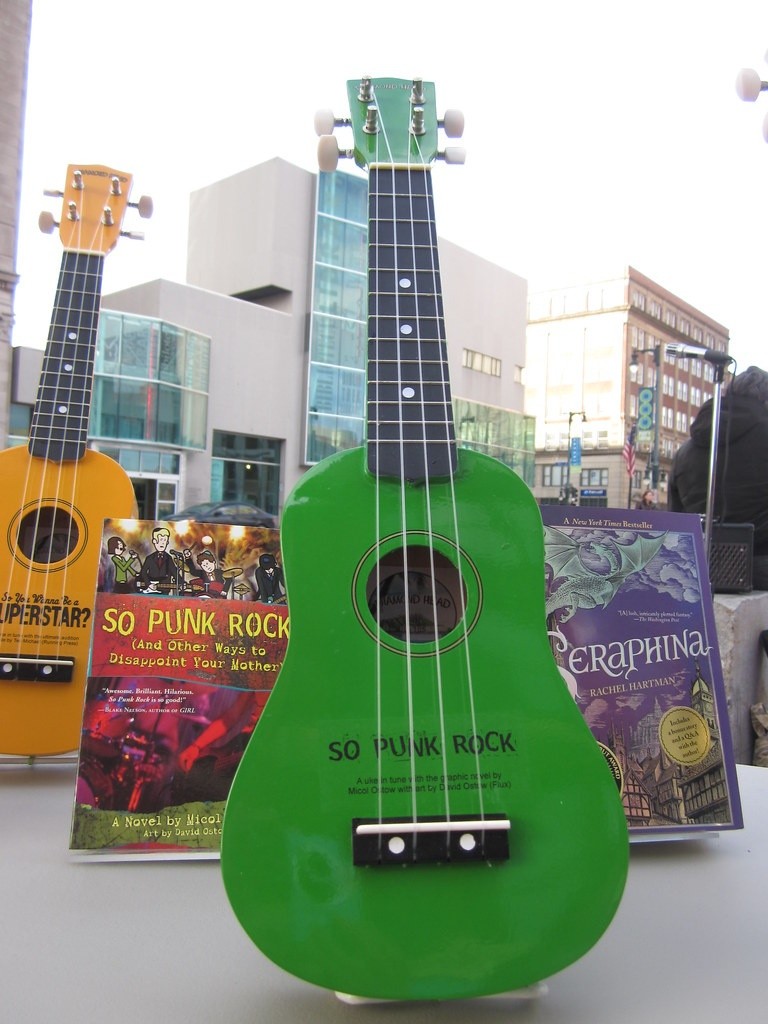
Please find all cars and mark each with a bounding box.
[160,501,275,528]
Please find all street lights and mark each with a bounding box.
[627,342,665,508]
[558,411,588,503]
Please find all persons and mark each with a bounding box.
[635,490,659,510]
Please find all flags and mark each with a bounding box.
[623,425,640,477]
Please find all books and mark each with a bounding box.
[538,505,745,845]
[65,518,289,863]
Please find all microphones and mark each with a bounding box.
[665,343,732,363]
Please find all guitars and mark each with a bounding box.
[215,76,632,1000]
[2,161,156,761]
